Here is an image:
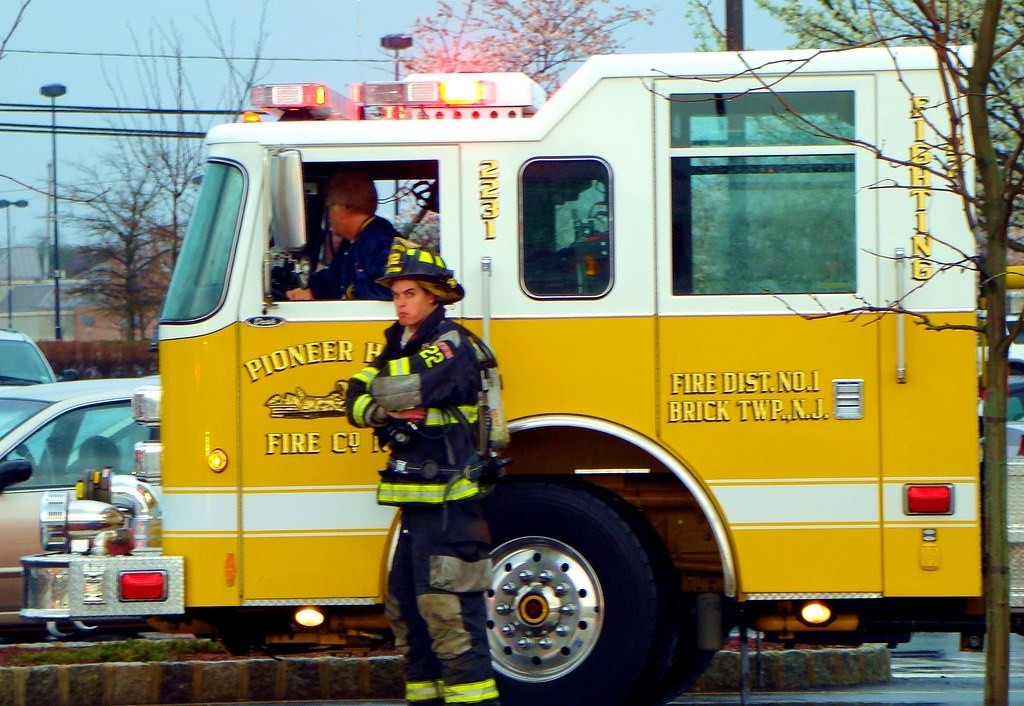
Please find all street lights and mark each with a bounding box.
[0,196,29,334]
[39,81,67,334]
[377,33,416,81]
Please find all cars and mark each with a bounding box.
[0,369,163,631]
[974,308,1024,459]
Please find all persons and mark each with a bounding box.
[268,174,400,300]
[23,435,120,484]
[345,240,502,706]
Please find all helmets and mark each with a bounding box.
[375,237,466,306]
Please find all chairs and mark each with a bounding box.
[68,435,124,471]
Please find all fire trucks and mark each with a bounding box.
[12,42,1024,706]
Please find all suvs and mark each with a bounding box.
[0,327,76,391]
